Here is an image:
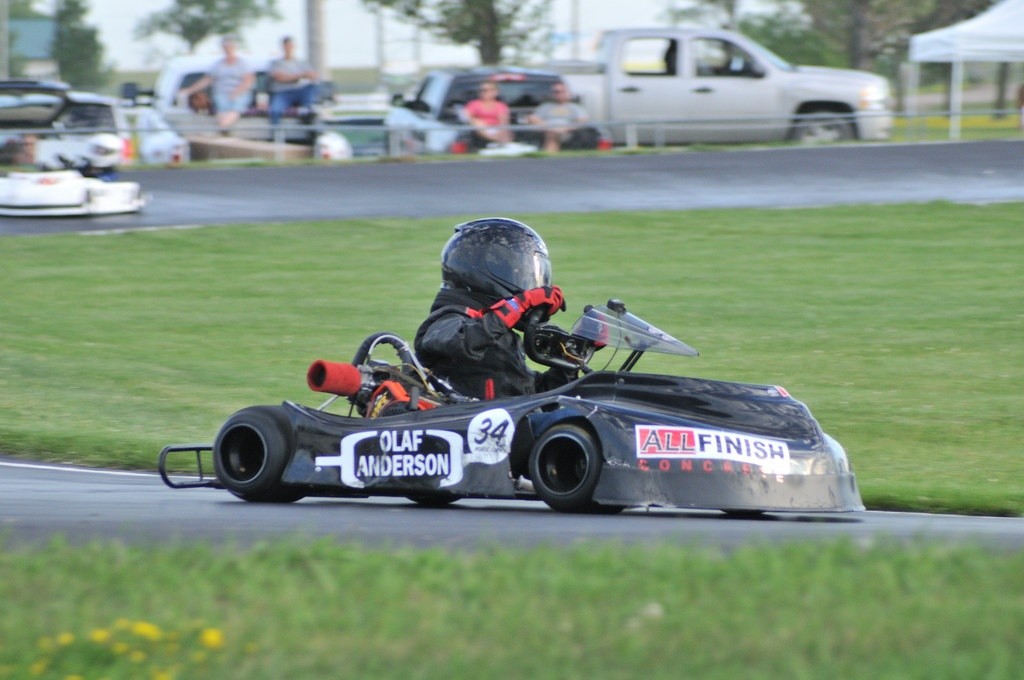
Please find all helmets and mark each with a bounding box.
[440,217,552,298]
[81,132,123,168]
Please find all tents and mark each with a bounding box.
[906,0,1024,141]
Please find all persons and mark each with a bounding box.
[458,82,510,154]
[414,219,608,402]
[0,134,66,177]
[529,82,588,152]
[72,135,122,183]
[266,36,322,143]
[179,34,257,137]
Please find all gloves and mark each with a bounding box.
[584,303,610,351]
[489,285,566,329]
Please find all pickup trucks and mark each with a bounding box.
[382,64,614,154]
[155,54,341,144]
[510,26,896,143]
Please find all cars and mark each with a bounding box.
[52,94,191,167]
